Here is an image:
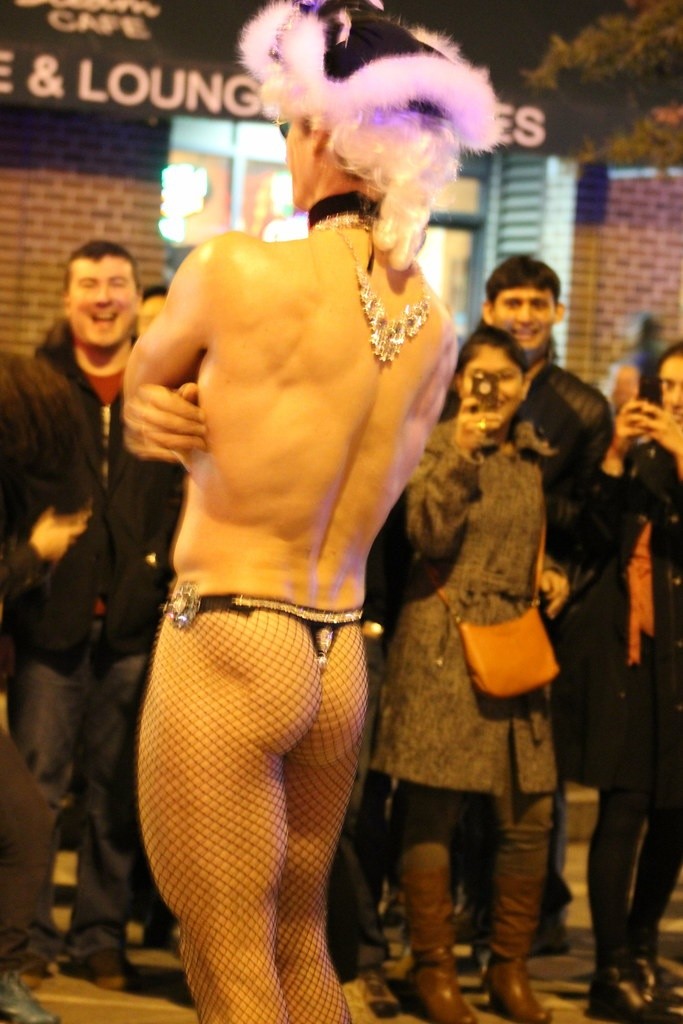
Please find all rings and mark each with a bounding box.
[480,419,486,431]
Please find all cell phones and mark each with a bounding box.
[636,374,664,420]
[470,369,500,413]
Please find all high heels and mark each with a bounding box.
[484,951,551,1024]
[413,948,480,1024]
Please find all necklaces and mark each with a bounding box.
[310,191,430,363]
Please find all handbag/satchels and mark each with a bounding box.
[455,602,561,700]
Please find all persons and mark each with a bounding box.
[0,239,682,1024]
[122,1,500,1024]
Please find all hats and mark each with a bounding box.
[238,0,512,269]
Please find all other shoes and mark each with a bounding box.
[70,950,140,991]
[627,950,666,1009]
[589,969,683,1024]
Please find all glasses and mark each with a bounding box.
[277,114,292,138]
